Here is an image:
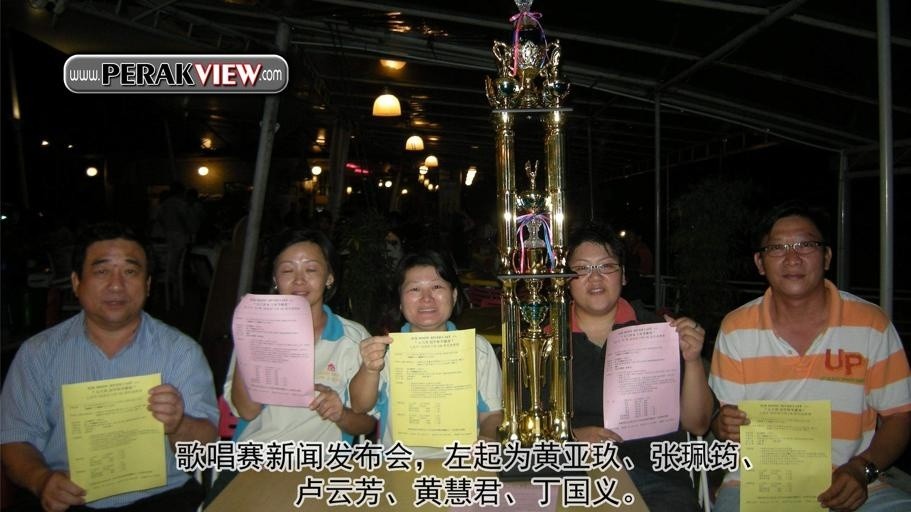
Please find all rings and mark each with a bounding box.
[693,321,698,331]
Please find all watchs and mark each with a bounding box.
[848,455,879,484]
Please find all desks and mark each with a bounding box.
[202,458,650,512]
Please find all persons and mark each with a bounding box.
[222,232,375,442]
[711,212,911,512]
[566,221,715,512]
[2,224,219,512]
[349,253,504,455]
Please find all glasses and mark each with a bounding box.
[570,263,624,277]
[759,240,824,257]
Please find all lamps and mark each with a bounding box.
[372,87,401,116]
